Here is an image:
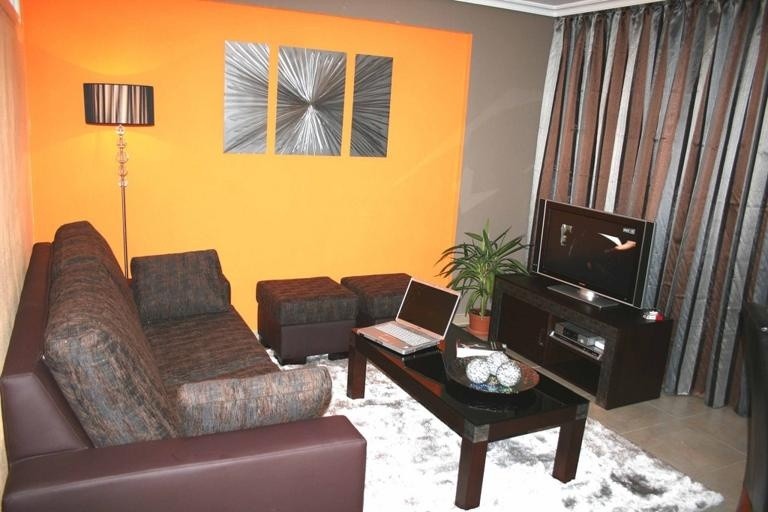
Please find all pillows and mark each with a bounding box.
[49,220,140,318]
[178,365,331,432]
[44,260,181,449]
[129,250,231,323]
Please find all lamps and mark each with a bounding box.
[83,82,155,277]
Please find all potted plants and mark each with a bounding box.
[435,220,528,340]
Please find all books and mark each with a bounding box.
[454,338,506,358]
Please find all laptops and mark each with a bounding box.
[357,280,462,356]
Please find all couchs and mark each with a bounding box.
[0,242,367,511]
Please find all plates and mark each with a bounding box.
[445,354,539,395]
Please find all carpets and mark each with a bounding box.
[251,324,723,512]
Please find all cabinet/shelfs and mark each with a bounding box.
[489,275,675,410]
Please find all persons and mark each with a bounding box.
[559,224,637,281]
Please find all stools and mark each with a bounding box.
[342,272,412,326]
[256,276,357,366]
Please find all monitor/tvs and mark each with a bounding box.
[531,198,658,310]
